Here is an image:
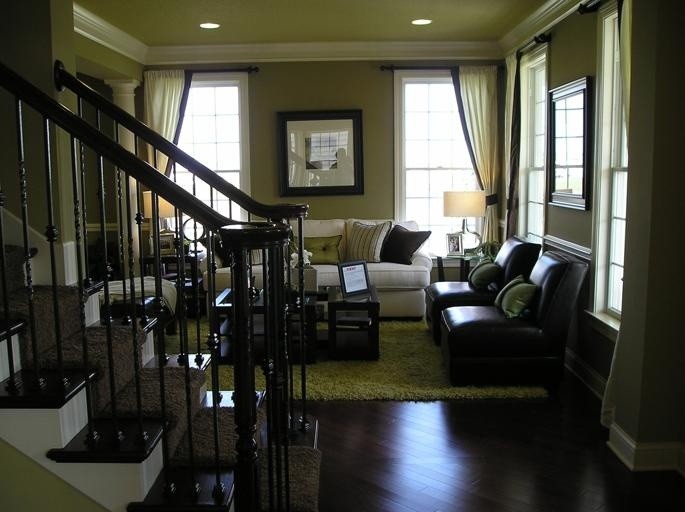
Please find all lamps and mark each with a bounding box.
[444,190,488,249]
[142,188,184,233]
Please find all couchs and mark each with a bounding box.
[201,218,432,318]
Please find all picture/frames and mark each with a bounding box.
[159,233,175,255]
[337,260,373,298]
[446,233,464,256]
[275,109,365,198]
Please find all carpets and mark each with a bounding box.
[154,317,550,401]
[1,243,322,510]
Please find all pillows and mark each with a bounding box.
[288,234,342,265]
[345,221,393,262]
[380,224,431,264]
[494,274,537,321]
[466,258,502,292]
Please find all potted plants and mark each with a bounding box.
[173,237,191,255]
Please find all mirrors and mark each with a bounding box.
[546,75,592,211]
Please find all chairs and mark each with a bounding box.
[423,235,541,346]
[440,250,590,387]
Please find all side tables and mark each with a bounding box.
[140,249,208,318]
[435,254,484,281]
